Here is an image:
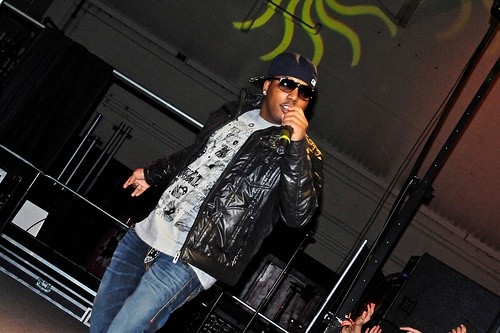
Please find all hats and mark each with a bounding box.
[248,51,318,90]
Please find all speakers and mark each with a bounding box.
[368,253,500,333]
[236,252,329,333]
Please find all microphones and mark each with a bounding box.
[275,124,294,155]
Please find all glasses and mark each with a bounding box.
[268,77,314,101]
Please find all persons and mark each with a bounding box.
[341,303,468,333]
[87,51,322,333]
